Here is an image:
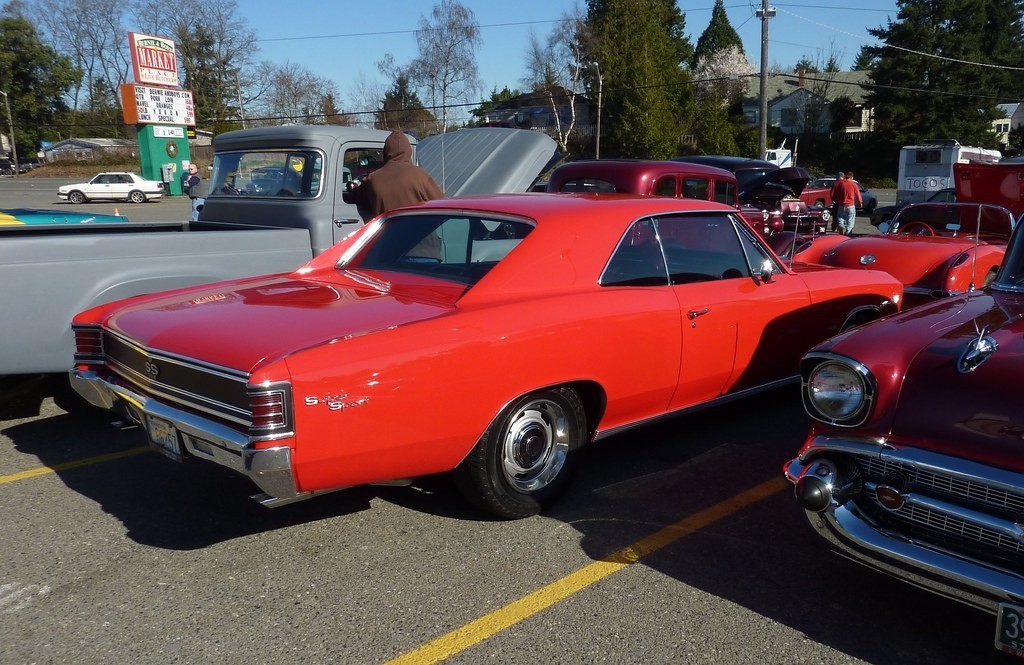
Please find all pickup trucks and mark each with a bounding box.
[0,125,560,430]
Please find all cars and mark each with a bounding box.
[811,177,879,213]
[69,193,906,520]
[782,211,1023,657]
[547,159,807,267]
[785,200,1017,309]
[57,172,164,204]
[0,163,29,175]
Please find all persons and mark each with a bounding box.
[357,130,449,265]
[831,171,864,236]
[184,165,203,219]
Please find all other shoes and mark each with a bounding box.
[839,226,846,235]
[833,226,839,233]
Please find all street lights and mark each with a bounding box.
[755,9,777,161]
